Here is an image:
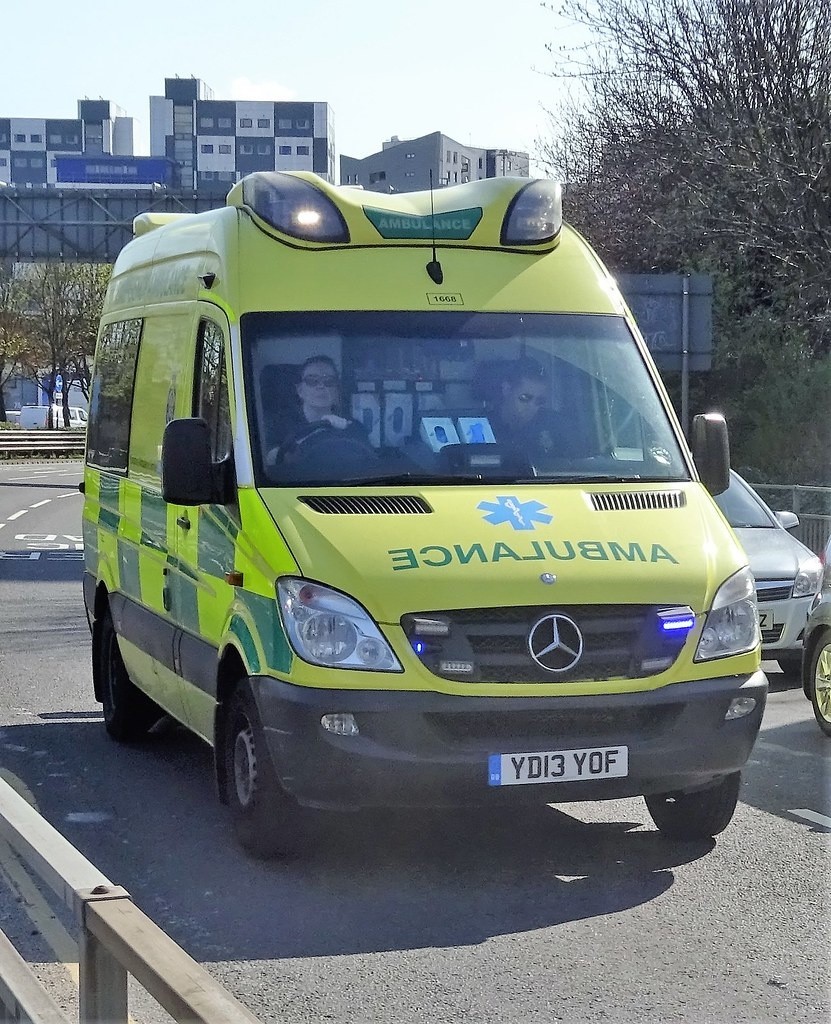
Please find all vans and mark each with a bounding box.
[20,404,88,429]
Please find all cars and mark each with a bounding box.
[800,528,831,737]
[689,451,824,680]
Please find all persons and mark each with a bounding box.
[262,353,375,466]
[479,359,578,461]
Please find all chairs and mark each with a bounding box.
[260,362,347,474]
[470,360,504,415]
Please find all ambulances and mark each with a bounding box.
[81,169,771,860]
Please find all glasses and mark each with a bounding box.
[519,393,546,406]
[301,373,336,387]
